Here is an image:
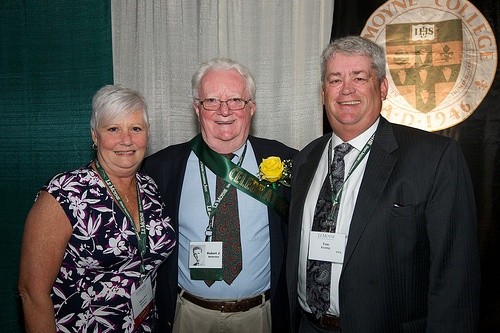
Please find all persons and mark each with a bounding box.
[19,84,178,333]
[193,246,204,266]
[137,58,300,333]
[285,35,476,332]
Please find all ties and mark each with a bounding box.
[306,144,354,320]
[212,154,243,286]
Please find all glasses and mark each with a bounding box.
[197,97,249,111]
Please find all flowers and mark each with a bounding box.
[257,156,293,188]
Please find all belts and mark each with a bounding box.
[176,285,270,312]
[304,312,342,328]
